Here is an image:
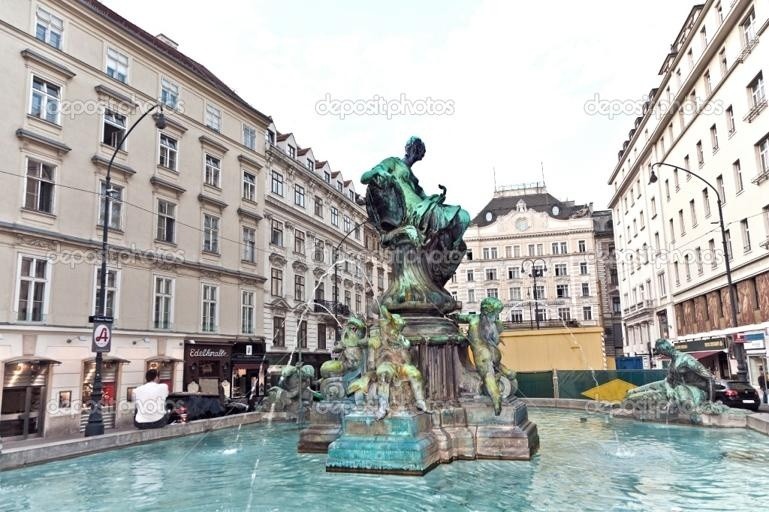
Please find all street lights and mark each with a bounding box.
[88,103,167,422]
[521,258,547,329]
[650,163,738,326]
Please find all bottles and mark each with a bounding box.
[176,401,188,423]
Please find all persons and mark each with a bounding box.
[361,135,462,246]
[765,372,769,395]
[758,366,769,403]
[374,314,426,421]
[479,297,504,368]
[131,369,178,429]
[312,319,366,386]
[625,339,722,415]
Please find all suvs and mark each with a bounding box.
[713,379,761,411]
